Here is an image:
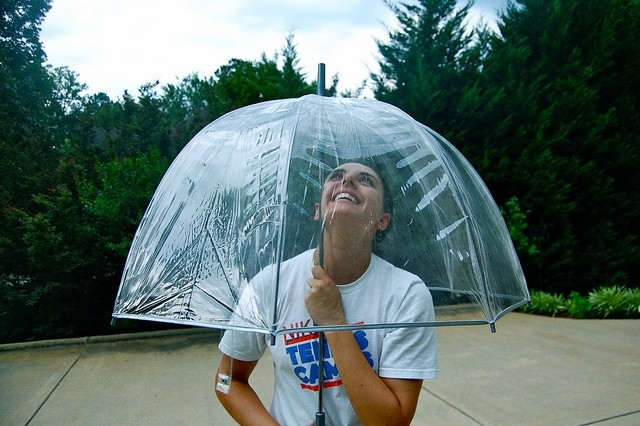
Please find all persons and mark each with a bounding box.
[215,162,438,425]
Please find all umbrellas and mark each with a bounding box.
[110,64,533,426]
